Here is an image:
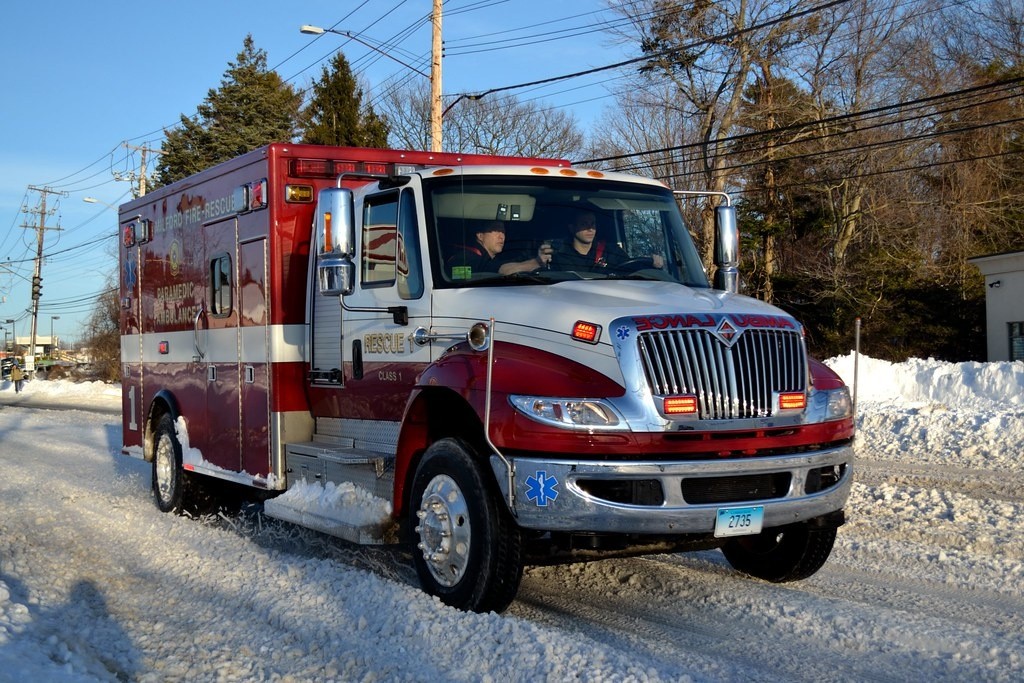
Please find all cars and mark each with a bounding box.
[35,365,53,380]
[22,366,37,379]
[70,363,99,381]
[1,357,20,376]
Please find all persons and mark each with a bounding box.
[548,211,664,271]
[444,220,553,278]
[11,360,24,394]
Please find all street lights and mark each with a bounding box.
[300,0,443,153]
[6,320,16,357]
[51,315,60,358]
[0,327,7,351]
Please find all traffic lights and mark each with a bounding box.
[31,276,43,300]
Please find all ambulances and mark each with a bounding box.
[118,138,856,616]
